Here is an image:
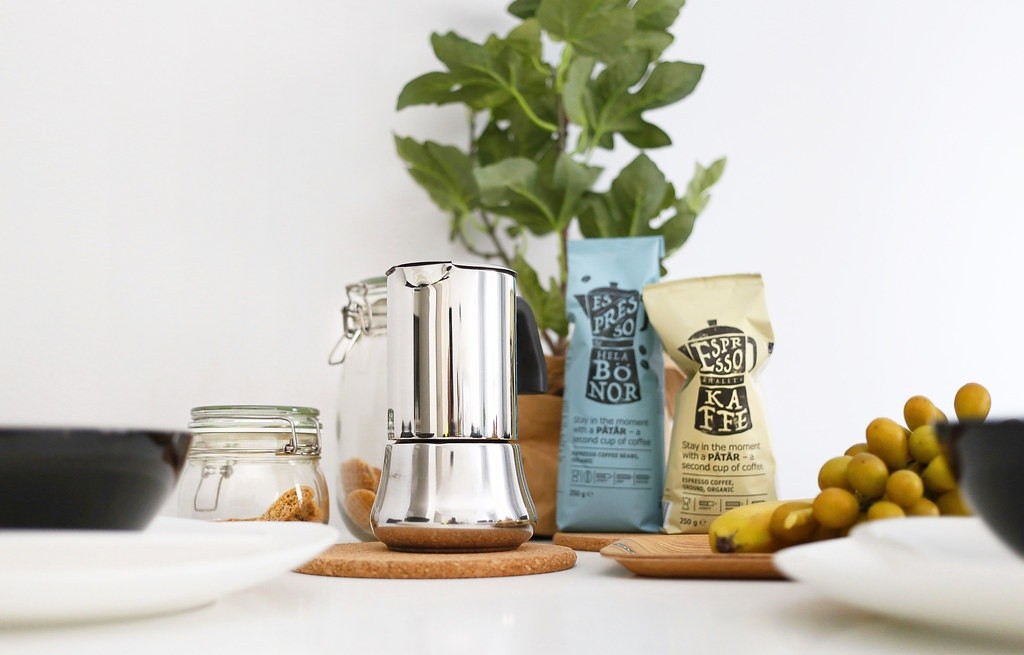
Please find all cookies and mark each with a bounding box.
[256,486,324,522]
[340,459,382,535]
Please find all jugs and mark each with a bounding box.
[369,262,554,549]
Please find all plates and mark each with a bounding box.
[772,516,1024,645]
[600,536,782,578]
[0,515,341,625]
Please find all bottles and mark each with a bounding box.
[174,404,331,527]
[327,274,388,535]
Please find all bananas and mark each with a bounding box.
[708,498,820,553]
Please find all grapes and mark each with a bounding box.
[768,382,992,541]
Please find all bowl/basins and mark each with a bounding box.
[932,417,1024,562]
[0,423,193,532]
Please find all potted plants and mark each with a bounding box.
[389,1,741,355]
[544,353,681,420]
